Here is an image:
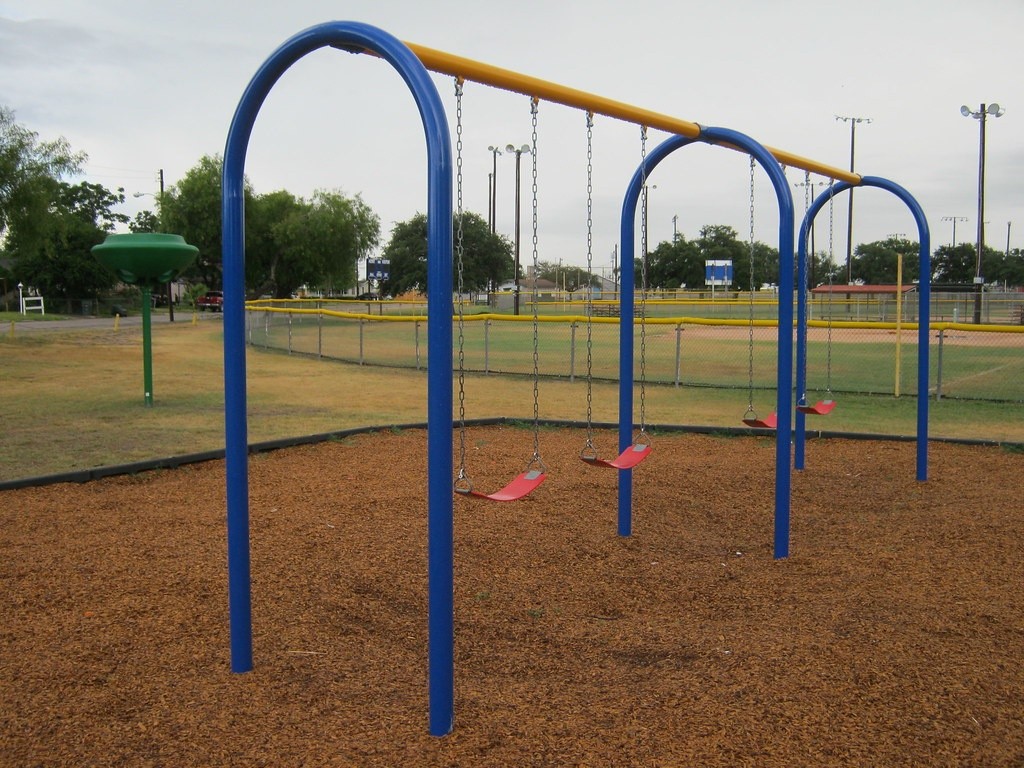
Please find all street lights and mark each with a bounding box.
[794,182,823,299]
[940,215,968,246]
[134,168,174,322]
[835,114,874,315]
[640,184,658,302]
[488,145,502,293]
[671,215,678,246]
[960,103,1006,327]
[506,143,531,316]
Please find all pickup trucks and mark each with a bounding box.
[196,291,225,313]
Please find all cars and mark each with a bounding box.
[354,292,379,301]
[150,294,168,309]
[112,304,127,317]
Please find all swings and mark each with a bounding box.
[577,128,653,473]
[740,167,777,428]
[794,183,838,416]
[453,88,547,502]
